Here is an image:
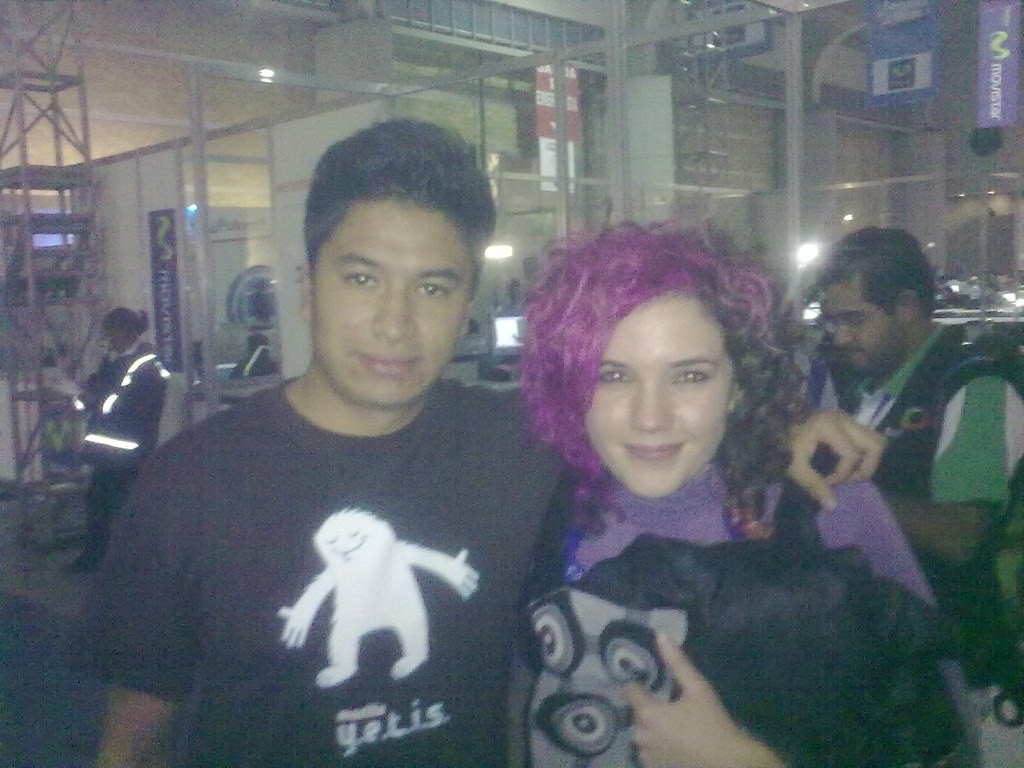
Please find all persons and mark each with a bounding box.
[75,120,1024,768]
